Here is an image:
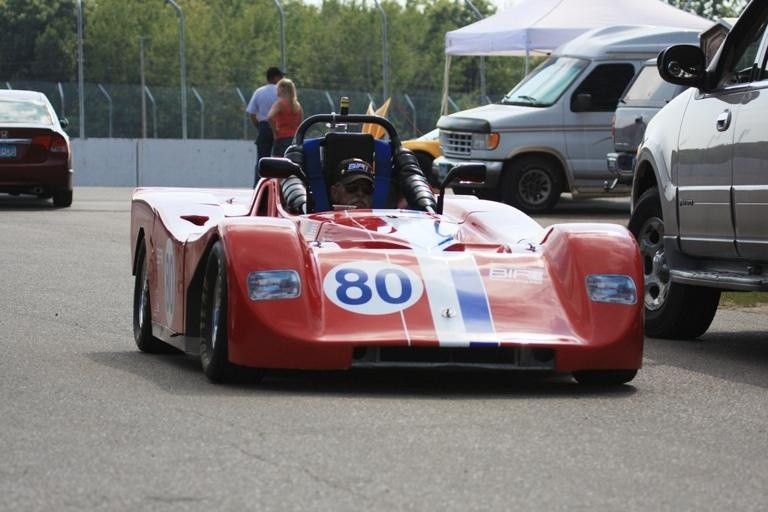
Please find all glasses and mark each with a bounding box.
[345,182,374,195]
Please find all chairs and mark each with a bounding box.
[302,132,396,211]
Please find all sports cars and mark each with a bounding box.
[128,96,644,390]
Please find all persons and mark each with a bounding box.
[329,157,378,210]
[248,66,286,189]
[265,76,304,159]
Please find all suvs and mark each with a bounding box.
[624,0,767,339]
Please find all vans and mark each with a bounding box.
[430,22,703,216]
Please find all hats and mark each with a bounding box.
[334,158,376,188]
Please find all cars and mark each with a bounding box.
[400,127,443,188]
[602,57,691,193]
[0,89,73,207]
